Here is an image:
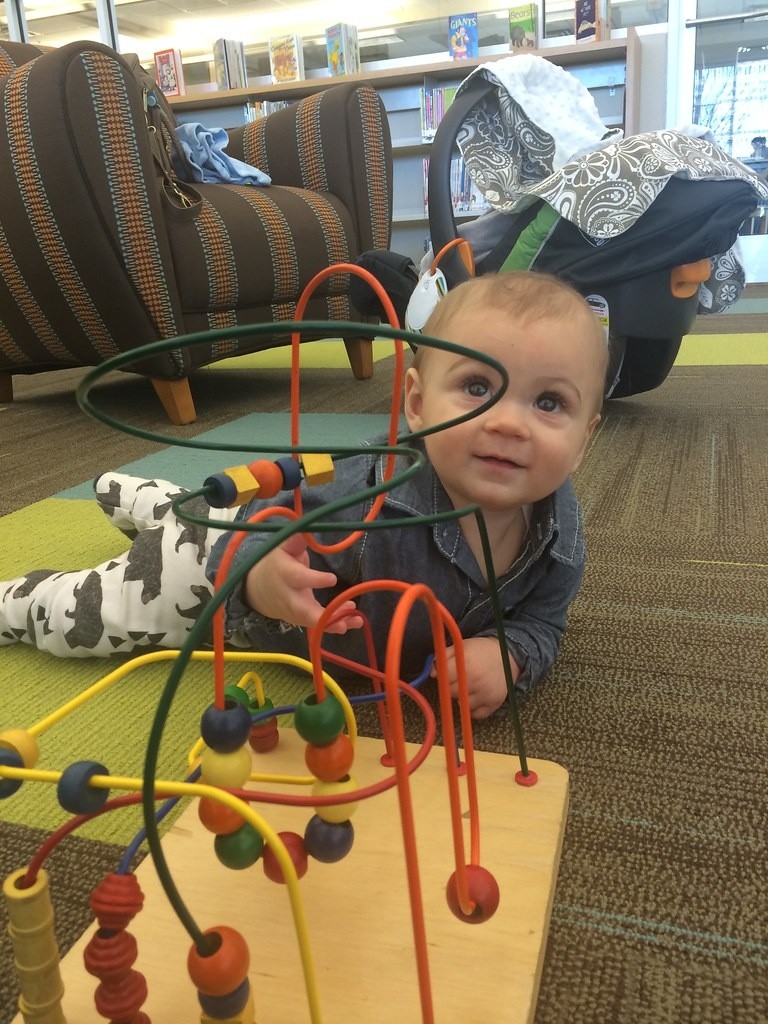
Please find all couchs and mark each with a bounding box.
[0,40,395,427]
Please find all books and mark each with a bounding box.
[154,0,614,220]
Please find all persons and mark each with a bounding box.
[1,271,608,720]
[749,136,768,159]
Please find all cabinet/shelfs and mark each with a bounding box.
[165,26,642,264]
